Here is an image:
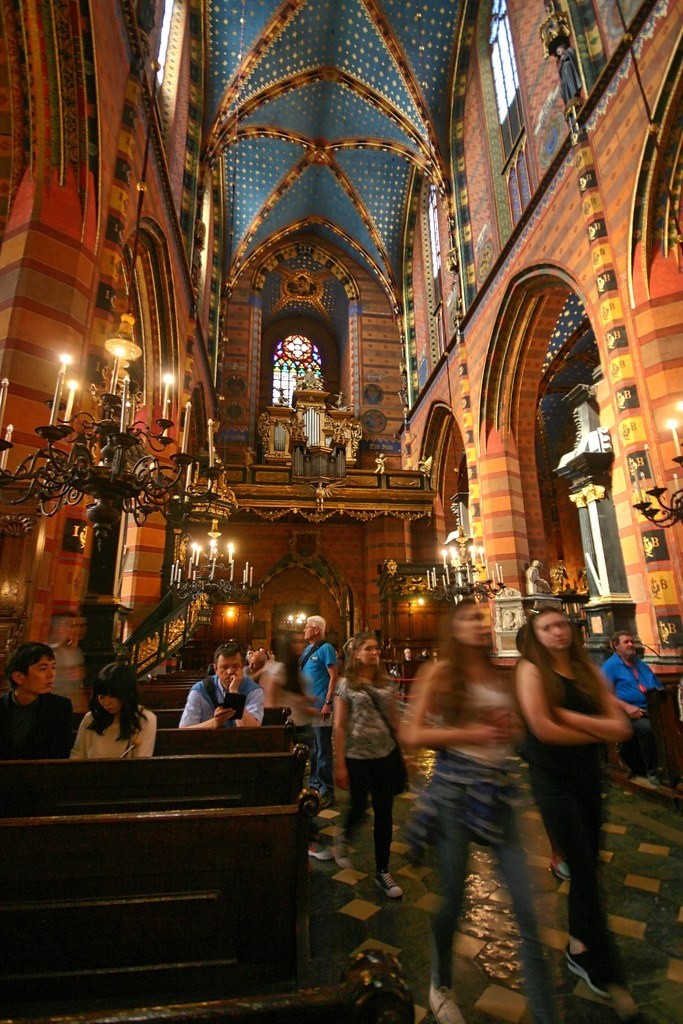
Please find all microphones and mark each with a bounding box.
[119,737,142,758]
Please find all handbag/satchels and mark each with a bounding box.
[394,752,410,793]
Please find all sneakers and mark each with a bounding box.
[334,844,353,868]
[375,872,403,896]
[565,945,613,997]
[308,845,330,859]
[319,794,334,808]
[429,985,465,1024]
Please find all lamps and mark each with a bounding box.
[168,0,262,606]
[0,57,225,552]
[425,126,507,610]
[631,401,683,528]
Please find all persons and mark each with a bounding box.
[242,446,256,471]
[602,630,663,785]
[514,607,634,1000]
[393,599,560,1024]
[0,641,73,760]
[242,615,338,810]
[418,455,431,477]
[179,643,265,728]
[332,632,402,898]
[374,453,387,473]
[404,647,430,661]
[69,662,158,759]
[276,388,347,442]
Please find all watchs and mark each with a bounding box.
[324,699,332,704]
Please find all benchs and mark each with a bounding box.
[0,669,415,1024]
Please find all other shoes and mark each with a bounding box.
[550,860,571,880]
